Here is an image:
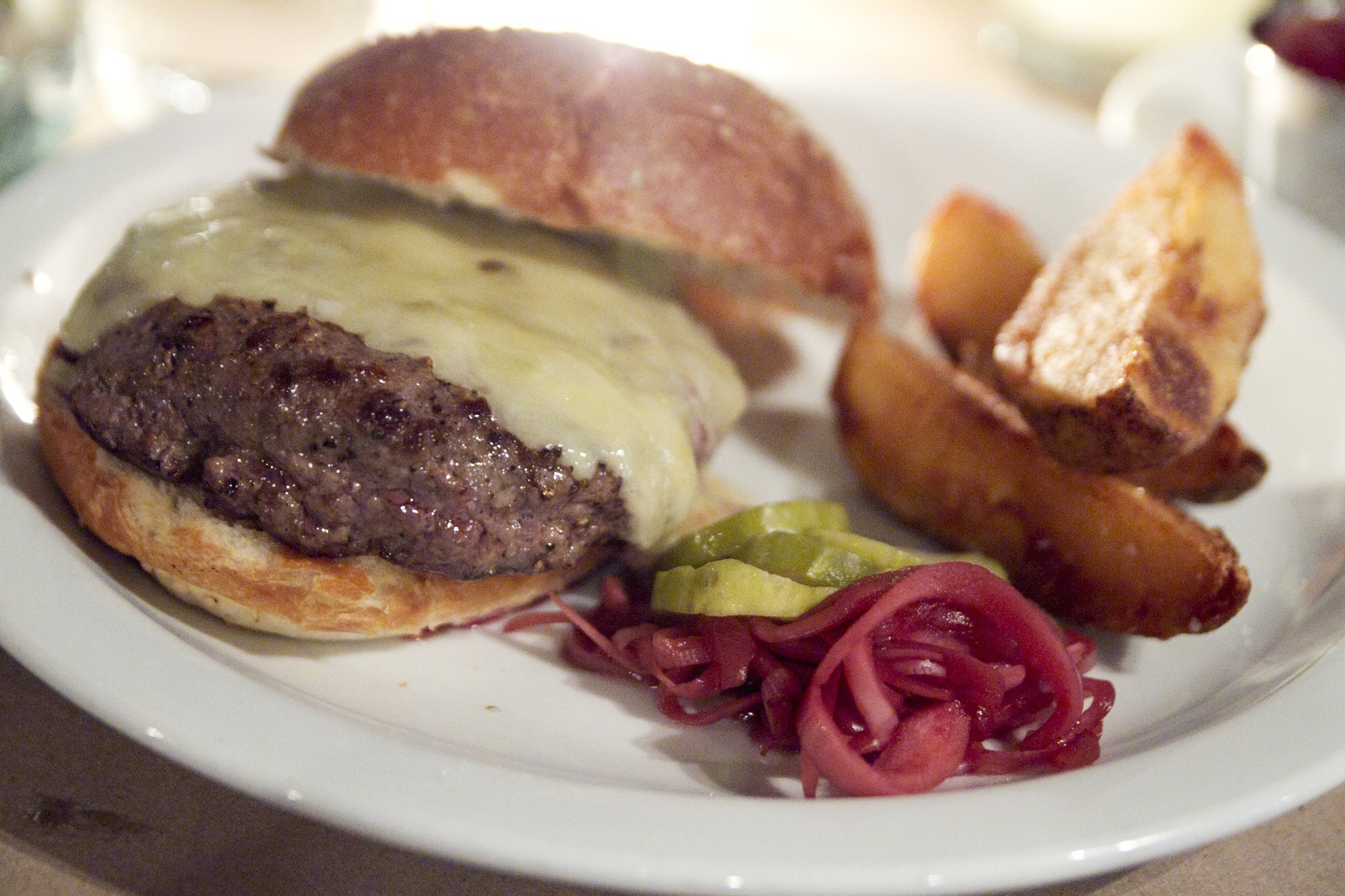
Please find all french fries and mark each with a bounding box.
[822,123,1270,646]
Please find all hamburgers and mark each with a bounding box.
[33,31,885,646]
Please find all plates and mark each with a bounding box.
[0,61,1345,894]
